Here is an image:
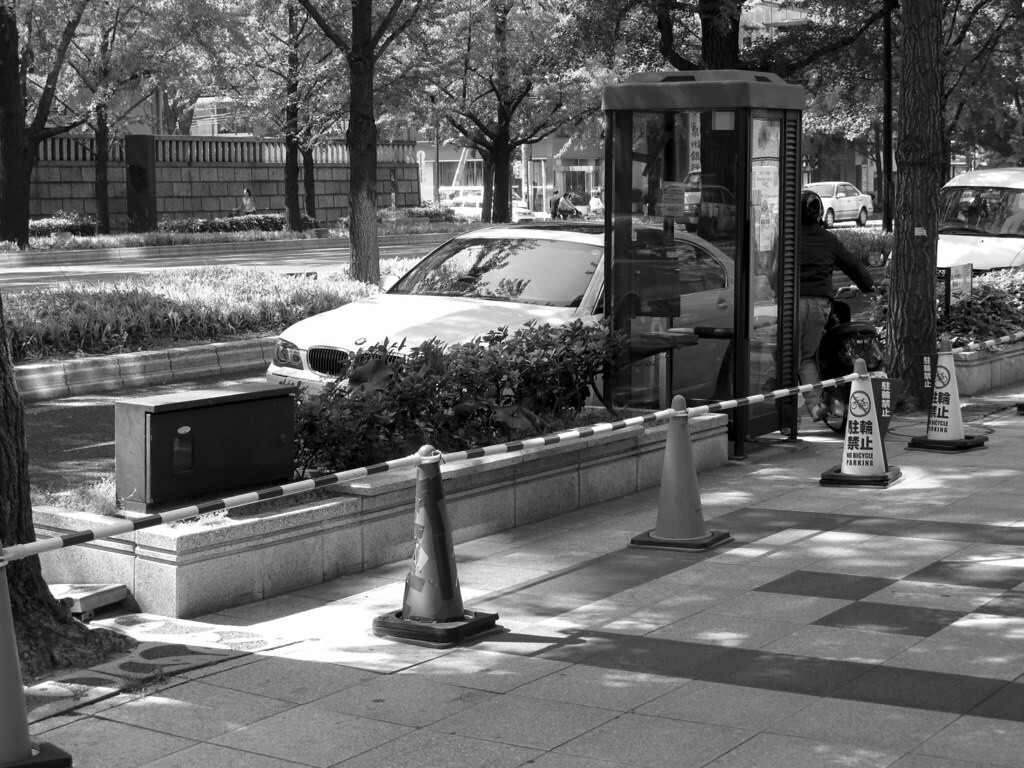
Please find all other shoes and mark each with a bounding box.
[810,402,830,422]
[780,428,791,435]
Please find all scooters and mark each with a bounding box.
[813,252,888,436]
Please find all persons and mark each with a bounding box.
[550,190,604,221]
[234,188,256,216]
[766,190,876,435]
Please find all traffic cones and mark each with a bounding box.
[818,359,902,489]
[373,444,504,649]
[627,395,734,552]
[1,544,73,767]
[904,339,989,454]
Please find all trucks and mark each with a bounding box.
[675,166,780,232]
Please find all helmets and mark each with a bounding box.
[801,189,824,219]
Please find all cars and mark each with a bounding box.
[885,168,1024,284]
[773,182,874,229]
[266,221,738,443]
[440,187,536,223]
